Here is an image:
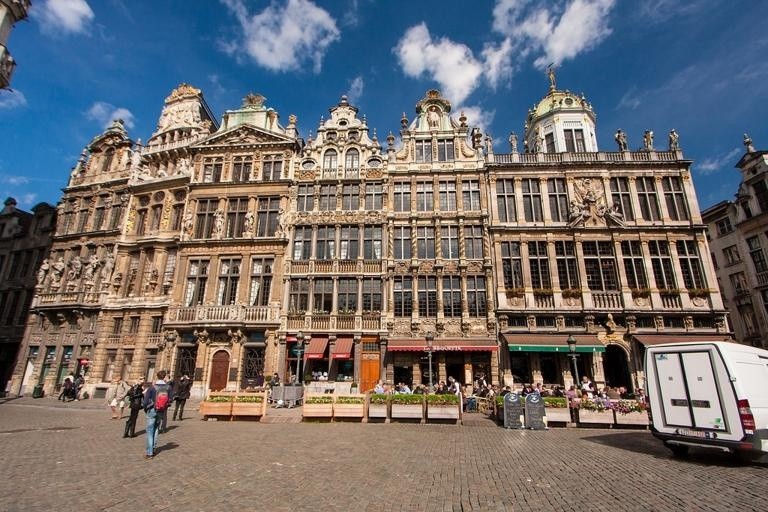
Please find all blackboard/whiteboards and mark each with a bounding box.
[504,393,524,429]
[525,393,547,430]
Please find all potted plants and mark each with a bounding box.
[496,395,571,422]
[301,394,461,423]
[350,382,357,394]
[198,395,264,418]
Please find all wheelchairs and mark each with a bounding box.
[62,382,77,401]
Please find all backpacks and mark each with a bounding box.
[124,383,137,410]
[153,384,169,410]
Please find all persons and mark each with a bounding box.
[106,370,193,460]
[58,372,86,402]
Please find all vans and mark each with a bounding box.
[643,341,768,467]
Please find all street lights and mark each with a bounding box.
[292,329,307,384]
[563,333,583,390]
[422,329,438,394]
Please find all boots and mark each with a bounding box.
[122,421,137,438]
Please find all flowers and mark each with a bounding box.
[573,397,646,416]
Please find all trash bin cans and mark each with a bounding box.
[32,384,42,398]
[272,382,303,408]
